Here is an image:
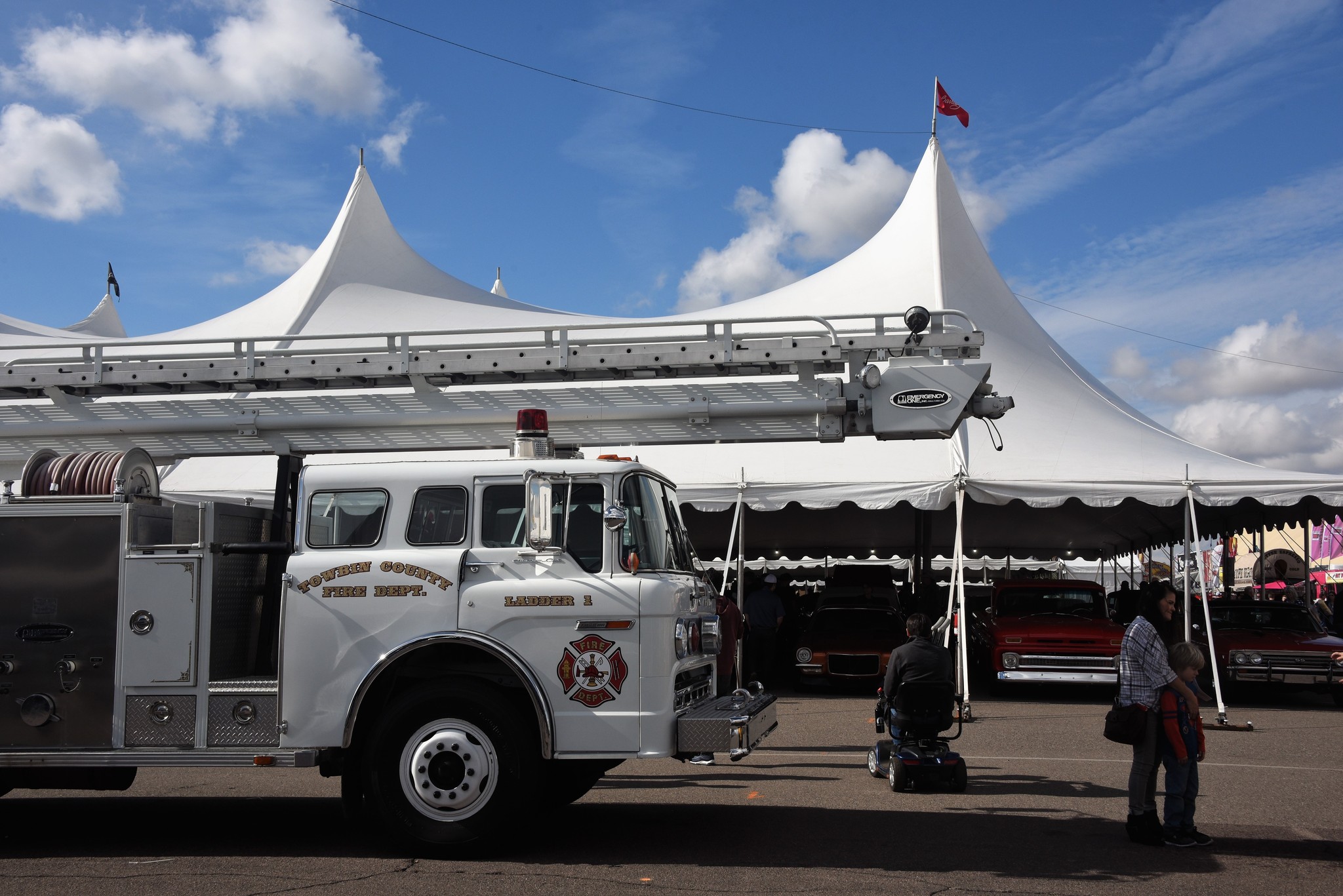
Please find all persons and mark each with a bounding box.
[708,571,940,689]
[1241,586,1343,638]
[1115,581,1170,631]
[1160,643,1213,847]
[883,613,953,745]
[1119,581,1199,847]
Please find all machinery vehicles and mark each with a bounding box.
[0,308,1018,844]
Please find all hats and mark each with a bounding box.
[1121,580,1129,587]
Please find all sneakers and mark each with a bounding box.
[1161,831,1197,847]
[690,754,715,766]
[747,672,757,689]
[1181,826,1214,846]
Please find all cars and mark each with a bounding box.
[715,563,1343,701]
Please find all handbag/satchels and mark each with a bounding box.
[1103,702,1147,745]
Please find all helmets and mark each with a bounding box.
[764,574,777,583]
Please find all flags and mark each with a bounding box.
[936,80,969,128]
[108,262,120,302]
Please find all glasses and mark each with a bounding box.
[1329,595,1335,596]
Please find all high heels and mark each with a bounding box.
[1144,810,1164,837]
[1125,814,1166,846]
